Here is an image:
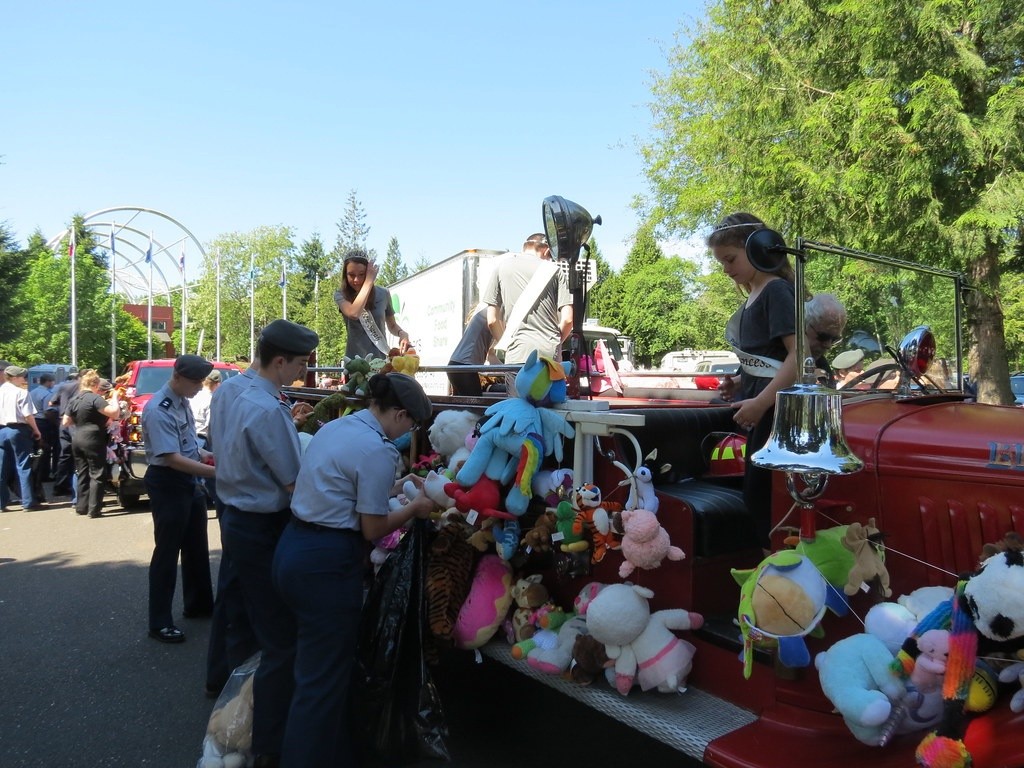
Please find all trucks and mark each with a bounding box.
[272,195,1024,768]
[384,247,636,397]
[659,351,735,388]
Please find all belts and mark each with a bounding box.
[290,513,352,533]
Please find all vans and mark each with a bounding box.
[694,358,742,389]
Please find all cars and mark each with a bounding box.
[1010,376,1024,405]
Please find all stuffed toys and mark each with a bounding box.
[370,348,704,698]
[730,516,1024,768]
[105,370,137,482]
[291,348,420,458]
[200,670,255,768]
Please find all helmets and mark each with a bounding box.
[704,433,747,477]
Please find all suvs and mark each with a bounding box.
[114,360,247,509]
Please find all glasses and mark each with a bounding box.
[394,407,421,432]
[809,324,842,344]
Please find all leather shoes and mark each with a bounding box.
[148,626,185,643]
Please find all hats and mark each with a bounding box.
[174,355,214,380]
[98,378,112,390]
[832,349,863,369]
[865,358,897,384]
[385,372,433,421]
[262,319,319,353]
[4,366,28,378]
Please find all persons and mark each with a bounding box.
[708,211,814,627]
[252,372,447,768]
[446,233,574,396]
[0,359,120,519]
[334,250,412,385]
[142,354,223,645]
[803,293,956,390]
[205,320,319,768]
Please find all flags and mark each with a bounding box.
[279,270,285,286]
[110,229,115,255]
[69,232,73,256]
[179,251,185,272]
[145,242,152,263]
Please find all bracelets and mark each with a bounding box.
[398,330,403,336]
